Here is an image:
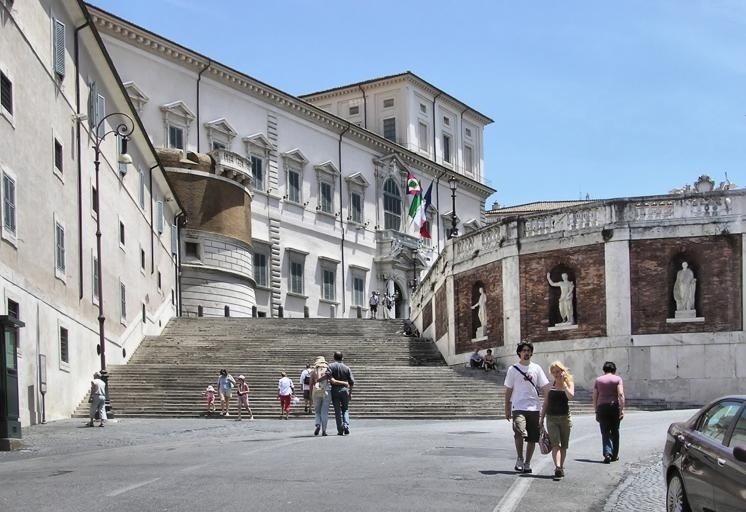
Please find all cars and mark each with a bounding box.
[661,391,746,512]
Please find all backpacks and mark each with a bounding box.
[304,375,312,384]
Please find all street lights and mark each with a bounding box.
[447,174,458,240]
[411,247,418,293]
[91,106,135,416]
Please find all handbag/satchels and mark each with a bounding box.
[537,426,554,454]
[230,381,235,388]
[312,387,329,400]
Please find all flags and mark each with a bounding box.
[414,181,433,239]
[408,179,427,228]
[406,173,423,197]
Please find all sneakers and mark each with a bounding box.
[207,409,254,421]
[313,425,327,436]
[87,423,104,427]
[604,453,619,463]
[553,468,568,481]
[337,424,350,436]
[515,458,533,473]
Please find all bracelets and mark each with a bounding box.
[314,378,317,383]
[562,385,569,392]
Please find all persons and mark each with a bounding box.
[470,285,488,338]
[277,371,294,419]
[309,356,350,436]
[369,291,379,319]
[300,363,313,414]
[85,371,107,427]
[401,323,416,336]
[217,368,236,416]
[592,360,627,462]
[674,259,699,318]
[201,385,217,415]
[484,347,498,373]
[470,347,483,368]
[547,271,576,326]
[236,374,254,420]
[381,292,392,319]
[503,339,550,473]
[312,351,354,434]
[538,359,575,478]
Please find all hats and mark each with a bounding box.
[206,385,215,391]
[315,356,327,365]
[238,375,245,381]
